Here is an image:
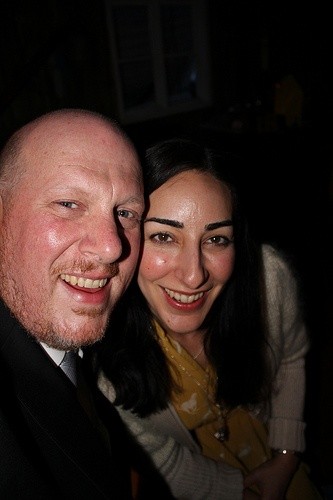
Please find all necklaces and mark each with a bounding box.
[148,317,234,443]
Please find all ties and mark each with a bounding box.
[62,352,78,389]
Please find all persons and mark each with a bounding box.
[0,108,178,500]
[95,137,321,500]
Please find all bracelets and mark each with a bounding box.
[274,447,297,457]
